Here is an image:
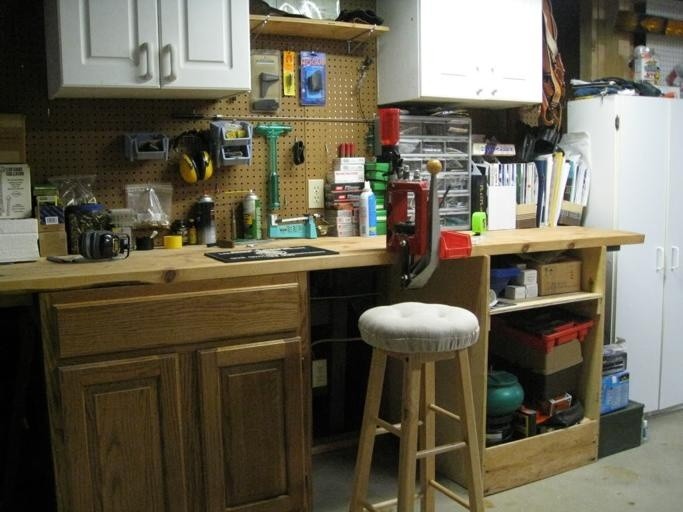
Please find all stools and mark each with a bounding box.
[348,302,486,512]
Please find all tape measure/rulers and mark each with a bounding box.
[472,212,487,236]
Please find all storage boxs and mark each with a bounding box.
[495,334,587,397]
[533,260,581,295]
[0,112,39,264]
[599,402,644,457]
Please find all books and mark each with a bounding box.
[471,134,591,232]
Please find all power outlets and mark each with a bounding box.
[307,179,324,209]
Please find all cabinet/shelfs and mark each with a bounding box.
[47,0,251,101]
[377,0,546,108]
[400,247,614,498]
[38,275,315,512]
[566,95,683,417]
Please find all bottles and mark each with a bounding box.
[175,218,196,245]
[380,108,399,145]
[634,45,656,87]
